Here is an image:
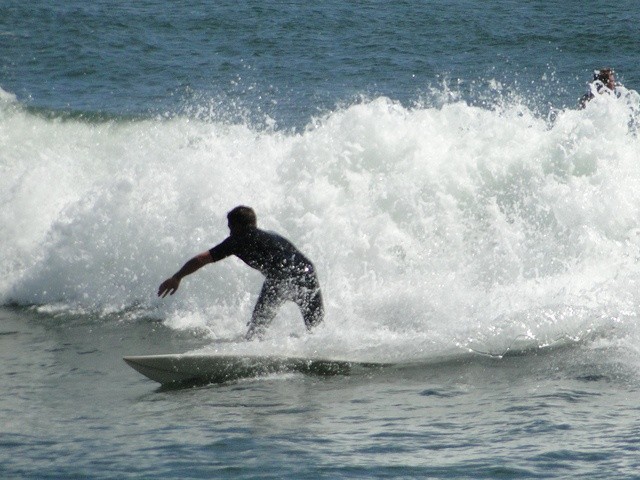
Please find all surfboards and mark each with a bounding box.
[122,355,397,383]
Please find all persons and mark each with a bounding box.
[155,204,328,341]
[579,67,618,108]
